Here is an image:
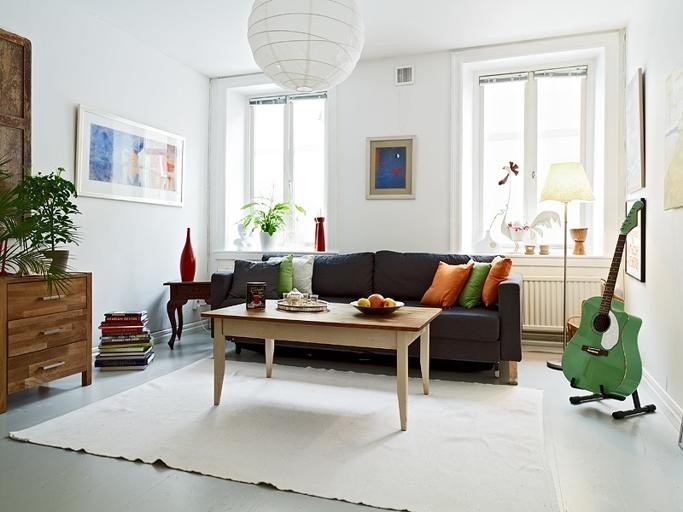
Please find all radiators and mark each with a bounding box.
[512,272,608,338]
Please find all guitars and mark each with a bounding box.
[562,201,646,397]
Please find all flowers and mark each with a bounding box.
[507,220,530,233]
[485,159,518,231]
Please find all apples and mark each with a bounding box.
[368,294,384,308]
[382,298,396,307]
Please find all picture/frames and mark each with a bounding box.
[622,195,646,284]
[361,134,419,202]
[71,103,186,211]
[622,65,646,196]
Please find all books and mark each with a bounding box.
[94,309,156,372]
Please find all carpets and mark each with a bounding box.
[5,337,681,512]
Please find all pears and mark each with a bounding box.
[357,298,370,307]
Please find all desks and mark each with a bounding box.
[162,281,215,350]
[196,292,442,434]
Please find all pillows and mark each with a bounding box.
[482,254,513,308]
[291,251,315,296]
[460,254,492,310]
[265,253,292,298]
[226,256,281,301]
[418,257,474,309]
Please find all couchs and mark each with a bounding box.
[206,250,526,385]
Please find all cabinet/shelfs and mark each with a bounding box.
[0,270,93,417]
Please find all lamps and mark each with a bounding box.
[538,160,598,372]
[244,0,364,94]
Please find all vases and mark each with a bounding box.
[473,229,499,256]
[509,229,527,242]
[313,215,325,251]
[178,226,198,282]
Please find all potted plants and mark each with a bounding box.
[235,194,309,255]
[17,163,83,273]
[13,171,54,277]
